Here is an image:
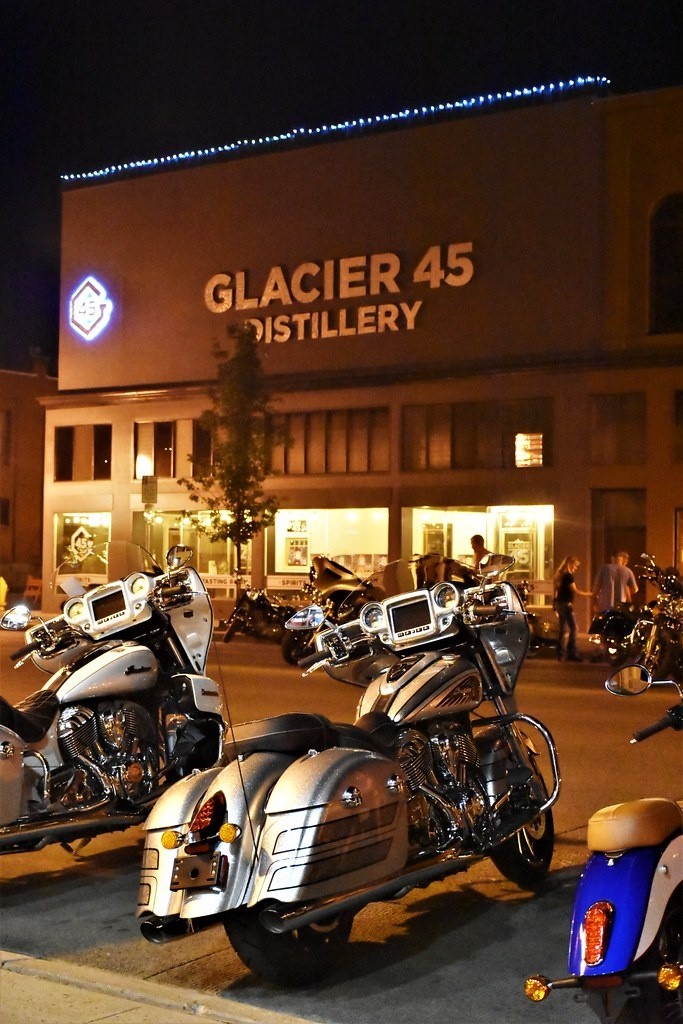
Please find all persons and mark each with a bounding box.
[594,551,639,611]
[552,554,593,662]
[470,534,493,569]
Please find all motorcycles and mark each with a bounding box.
[630,552,683,677]
[282,554,363,665]
[133,553,559,984]
[0,544,230,856]
[524,664,683,1024]
[223,568,298,643]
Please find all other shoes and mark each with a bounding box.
[567,656,583,662]
[557,654,565,663]
